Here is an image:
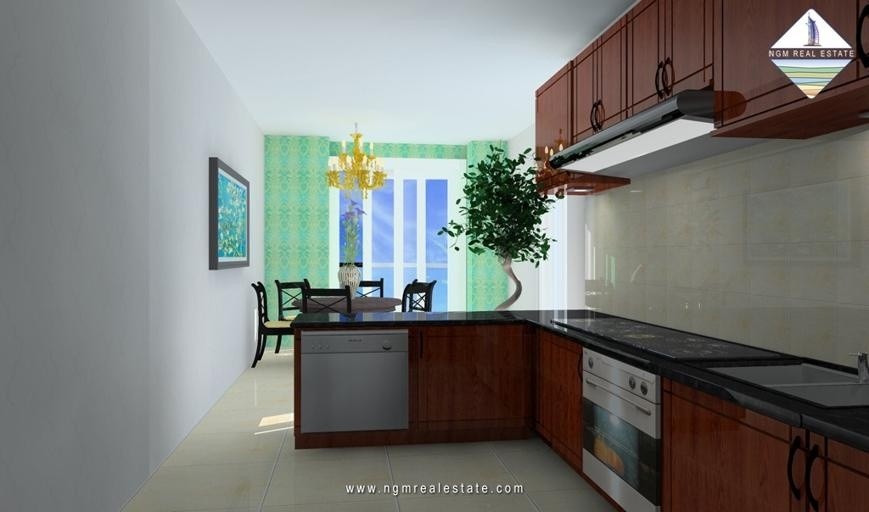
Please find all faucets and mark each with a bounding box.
[847,351,869,383]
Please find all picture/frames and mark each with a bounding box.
[208,156,250,270]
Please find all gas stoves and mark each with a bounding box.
[550,316,782,360]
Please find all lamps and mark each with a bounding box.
[323,123,386,200]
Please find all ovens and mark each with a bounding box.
[579,346,661,512]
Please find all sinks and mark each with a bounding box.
[762,384,869,408]
[706,356,862,385]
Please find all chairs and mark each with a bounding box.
[250,278,437,369]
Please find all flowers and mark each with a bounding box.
[341,200,367,264]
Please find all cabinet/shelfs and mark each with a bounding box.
[528,322,582,473]
[659,378,869,512]
[711,0,869,139]
[572,0,715,160]
[535,60,631,196]
[413,324,532,445]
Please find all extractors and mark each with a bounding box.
[545,89,714,174]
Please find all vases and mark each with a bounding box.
[338,264,361,301]
